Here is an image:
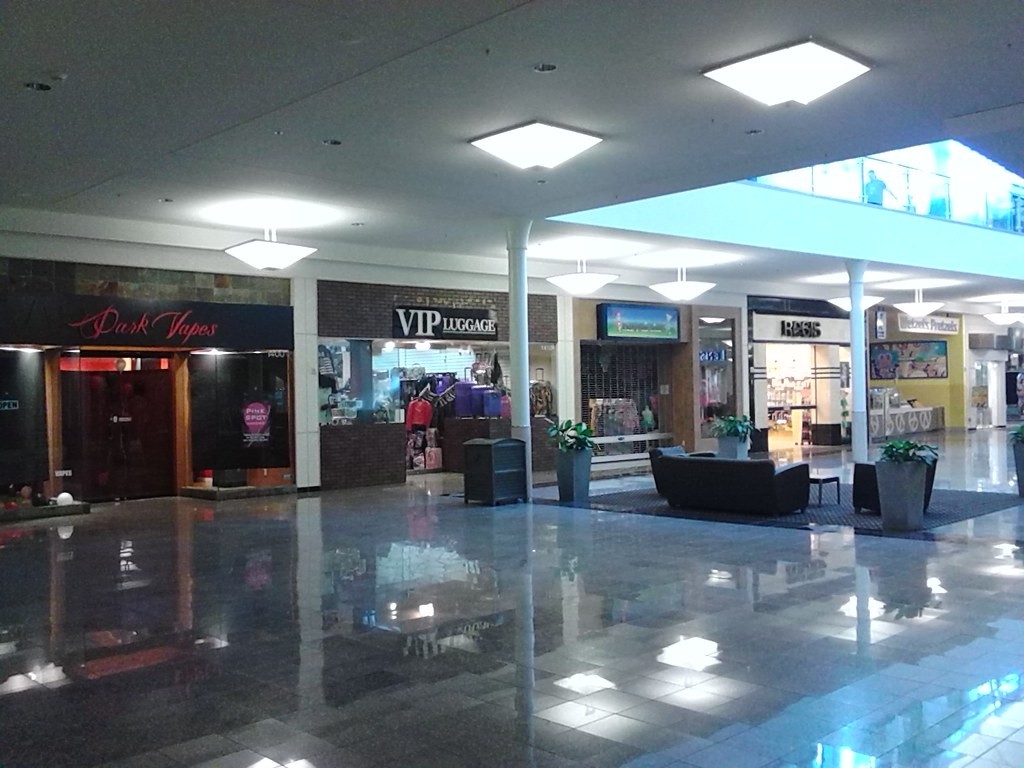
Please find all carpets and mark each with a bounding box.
[537,483,1024,541]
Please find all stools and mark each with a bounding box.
[809,476,841,506]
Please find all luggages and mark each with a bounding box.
[501,375,513,418]
[471,370,495,419]
[529,368,552,418]
[453,365,478,419]
[482,387,501,420]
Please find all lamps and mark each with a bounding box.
[468,121,603,171]
[982,306,1024,325]
[546,260,618,297]
[891,288,944,321]
[700,34,881,107]
[648,268,717,302]
[221,228,319,269]
[826,296,884,312]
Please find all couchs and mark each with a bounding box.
[649,446,685,467]
[655,451,810,518]
[852,454,937,513]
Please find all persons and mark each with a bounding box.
[864,169,898,207]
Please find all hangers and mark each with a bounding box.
[414,387,456,407]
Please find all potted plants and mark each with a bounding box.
[709,414,754,459]
[875,440,939,528]
[547,419,596,502]
[1008,424,1024,497]
